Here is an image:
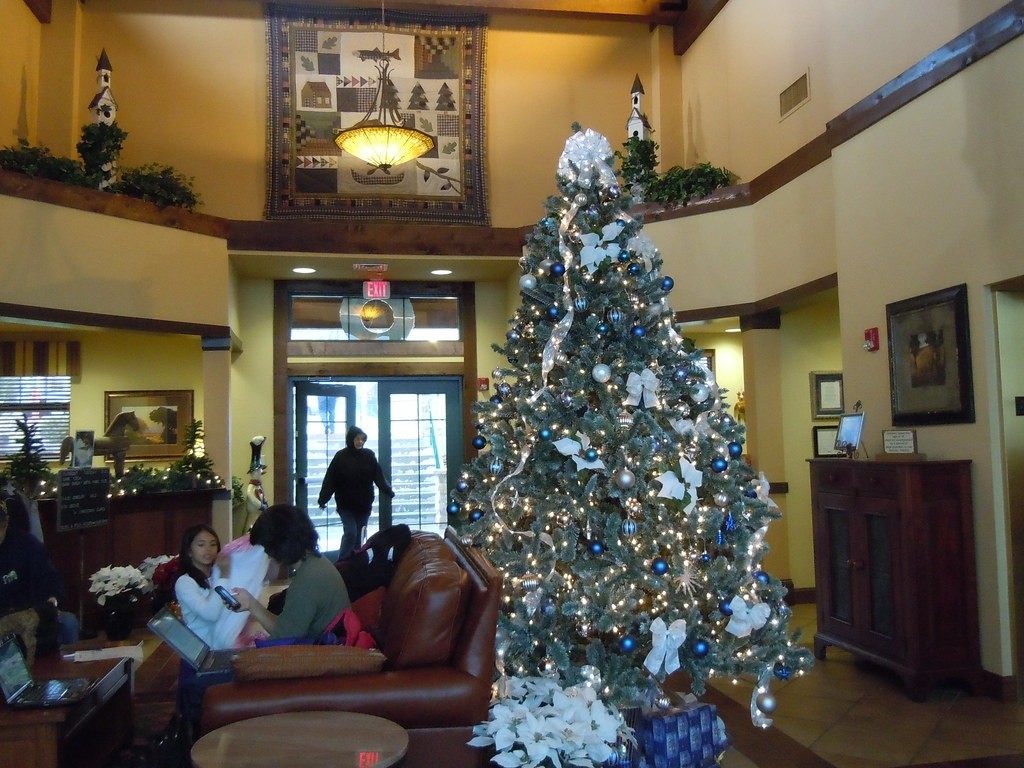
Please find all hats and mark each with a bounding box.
[250,504,314,545]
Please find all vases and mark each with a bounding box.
[101,594,132,641]
[147,589,171,617]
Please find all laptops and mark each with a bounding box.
[0,633,99,708]
[145,606,250,678]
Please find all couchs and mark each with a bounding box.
[204,525,507,729]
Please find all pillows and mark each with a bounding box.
[234,644,386,681]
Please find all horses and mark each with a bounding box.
[58,410,139,478]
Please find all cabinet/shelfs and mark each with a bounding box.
[803,458,983,702]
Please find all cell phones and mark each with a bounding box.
[214,586,241,610]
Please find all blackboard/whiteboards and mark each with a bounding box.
[56,467,110,532]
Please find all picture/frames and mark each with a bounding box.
[884,283,976,425]
[813,425,848,457]
[810,371,845,420]
[102,390,197,459]
[834,412,866,450]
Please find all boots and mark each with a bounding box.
[131,714,200,768]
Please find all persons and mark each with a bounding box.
[0,499,80,643]
[317,426,395,560]
[169,524,230,740]
[222,505,352,639]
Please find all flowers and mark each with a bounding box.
[467,676,636,768]
[137,552,181,591]
[91,565,144,607]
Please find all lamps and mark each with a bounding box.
[334,1,435,169]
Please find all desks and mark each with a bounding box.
[0,639,144,768]
[191,709,410,767]
